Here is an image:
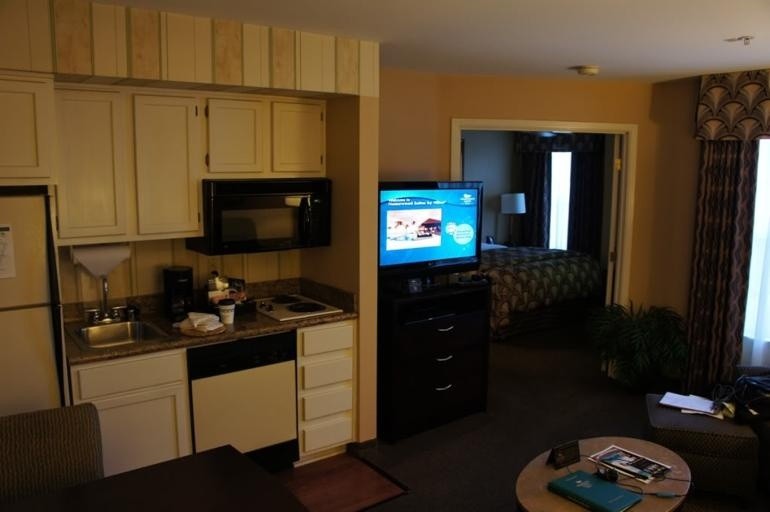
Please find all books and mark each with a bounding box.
[588,444,671,485]
[721,400,759,418]
[659,391,717,413]
[547,470,641,512]
[681,394,725,420]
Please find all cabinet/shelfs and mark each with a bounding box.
[70,319,358,478]
[204,90,326,178]
[1,70,54,186]
[378,276,493,437]
[53,81,204,248]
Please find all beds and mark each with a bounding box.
[432,243,603,341]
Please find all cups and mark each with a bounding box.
[217,298,236,324]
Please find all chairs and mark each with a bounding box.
[0,402,103,498]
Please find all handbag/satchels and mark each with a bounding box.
[734,375,770,423]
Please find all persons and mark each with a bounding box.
[387,220,418,242]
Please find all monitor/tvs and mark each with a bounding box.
[378,180,484,292]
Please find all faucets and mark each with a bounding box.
[102,277,109,317]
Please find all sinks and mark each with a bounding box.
[80,320,170,348]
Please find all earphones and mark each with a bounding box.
[591,462,696,498]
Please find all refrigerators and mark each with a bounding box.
[0,185,71,417]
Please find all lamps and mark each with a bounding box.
[502,193,526,247]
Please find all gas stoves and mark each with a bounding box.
[248,293,344,322]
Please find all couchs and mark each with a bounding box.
[647,364,770,505]
